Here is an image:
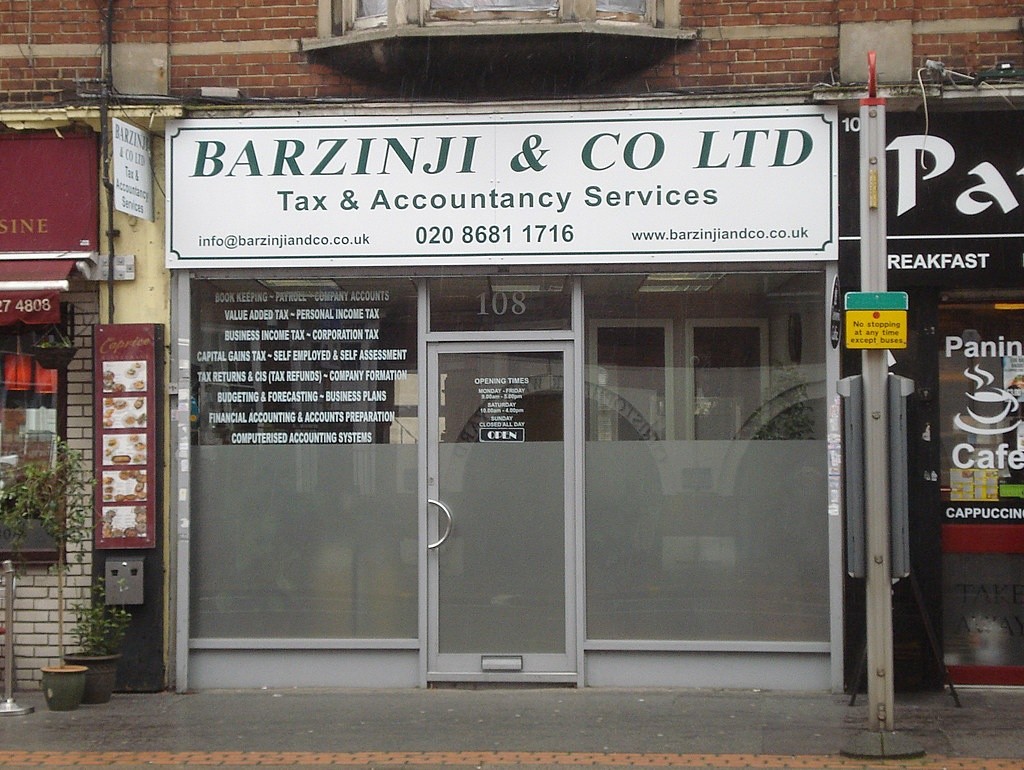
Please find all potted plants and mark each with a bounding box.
[35,335,77,370]
[62,577,132,705]
[0,436,111,712]
